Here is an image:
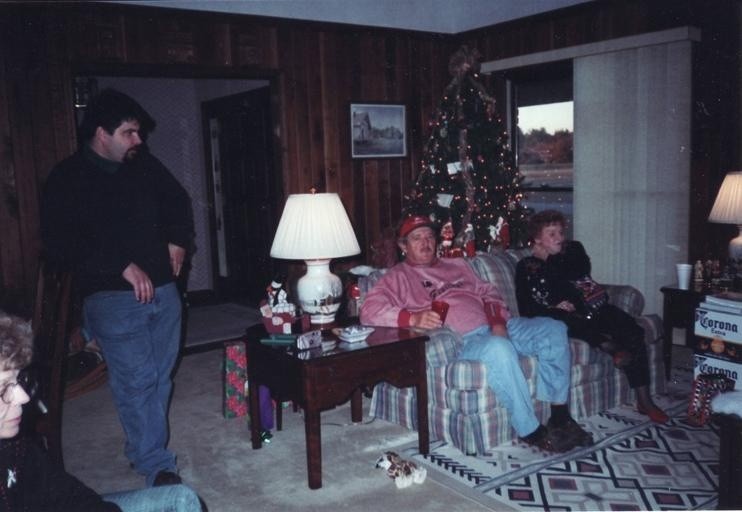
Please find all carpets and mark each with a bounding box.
[356,383,718,509]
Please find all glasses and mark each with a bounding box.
[1,370,30,404]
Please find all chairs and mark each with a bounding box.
[13,254,72,471]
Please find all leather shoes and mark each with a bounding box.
[547,414,593,446]
[156,472,180,484]
[536,423,576,455]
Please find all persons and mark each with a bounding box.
[0,312,204,510]
[513,209,671,423]
[40,89,201,489]
[359,215,594,452]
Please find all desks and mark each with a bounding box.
[658,282,705,380]
[243,324,430,490]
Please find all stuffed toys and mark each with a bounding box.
[373,451,428,489]
[267,273,295,314]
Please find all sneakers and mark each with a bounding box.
[614,351,633,367]
[637,403,668,422]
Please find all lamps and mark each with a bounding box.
[269,186,361,331]
[707,170,741,293]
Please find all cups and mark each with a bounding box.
[432,300,450,325]
[677,264,692,290]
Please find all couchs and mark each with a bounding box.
[706,391,742,510]
[358,246,668,456]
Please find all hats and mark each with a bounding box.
[400,214,435,235]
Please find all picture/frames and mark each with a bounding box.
[348,100,411,161]
